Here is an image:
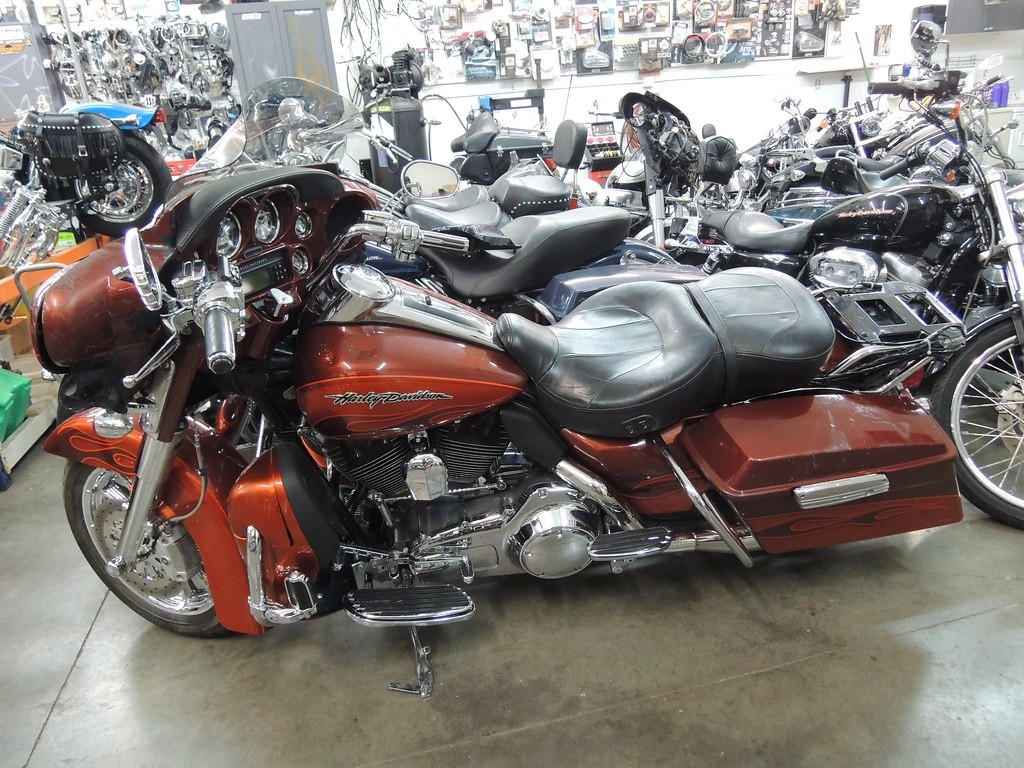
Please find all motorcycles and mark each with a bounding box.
[14,20,1024,701]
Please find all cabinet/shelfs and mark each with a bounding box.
[224,0,343,122]
[967,108,1024,172]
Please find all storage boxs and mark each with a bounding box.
[0,336,32,445]
